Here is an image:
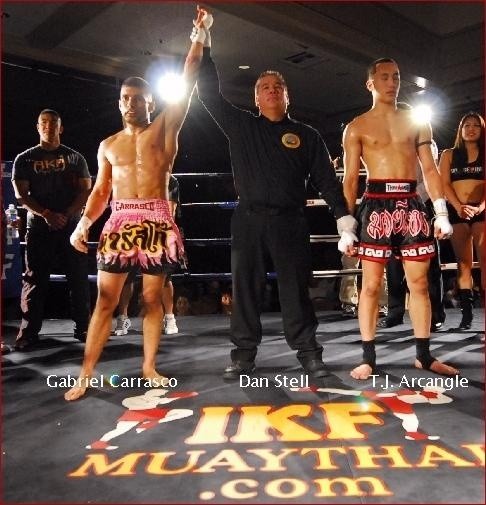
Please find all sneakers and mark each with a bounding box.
[342,304,355,317]
[305,359,330,378]
[14,334,39,350]
[114,316,131,335]
[379,305,388,316]
[163,314,179,334]
[223,360,256,380]
[459,322,471,329]
[73,328,87,341]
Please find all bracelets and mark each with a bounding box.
[40,208,51,218]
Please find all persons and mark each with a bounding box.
[373,100,447,332]
[331,120,390,318]
[331,56,461,379]
[439,111,486,343]
[114,173,182,336]
[86,386,200,452]
[10,108,94,351]
[188,15,360,380]
[289,383,455,442]
[133,275,481,317]
[63,4,215,403]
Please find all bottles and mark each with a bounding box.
[6,205,20,241]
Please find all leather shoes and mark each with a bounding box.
[430,321,443,330]
[381,317,403,328]
